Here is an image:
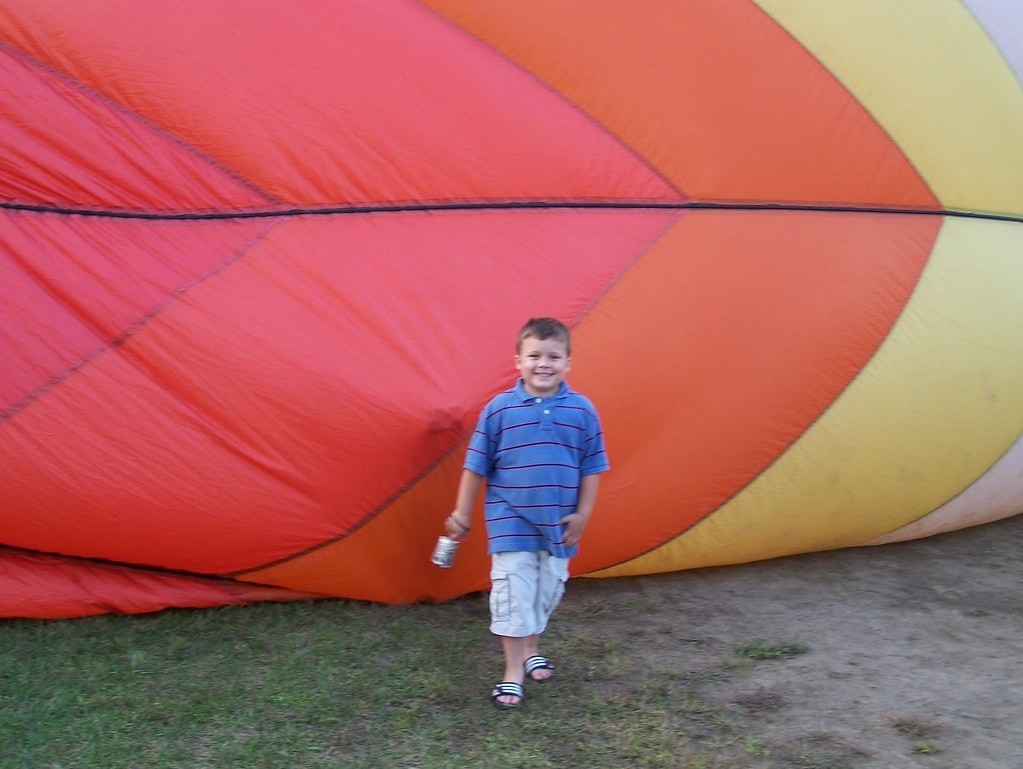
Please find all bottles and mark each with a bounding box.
[430,528,461,570]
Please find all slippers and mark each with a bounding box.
[492,681,524,709]
[524,655,554,683]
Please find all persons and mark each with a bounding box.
[445,317,610,711]
